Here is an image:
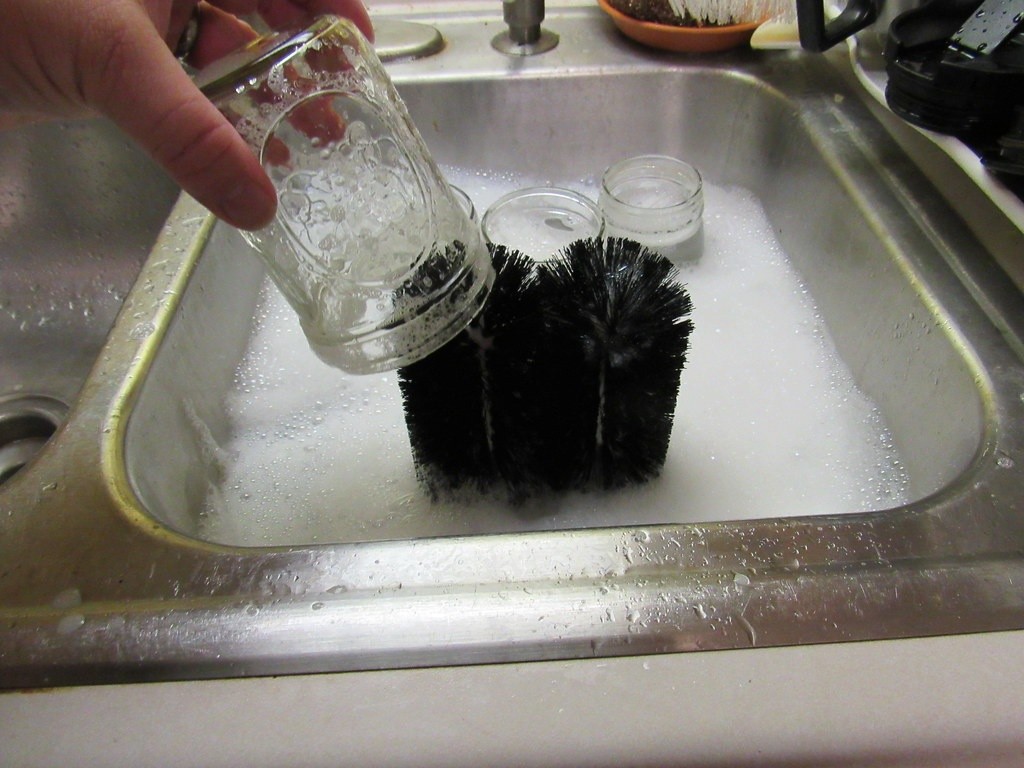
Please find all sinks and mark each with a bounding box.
[98,61,1002,556]
[0,107,187,498]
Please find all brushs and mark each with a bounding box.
[393,243,690,497]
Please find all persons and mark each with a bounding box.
[0,0,376,231]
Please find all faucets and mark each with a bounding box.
[488,0,560,58]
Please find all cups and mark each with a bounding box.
[597,154,704,264]
[446,182,480,232]
[193,14,497,375]
[481,185,606,281]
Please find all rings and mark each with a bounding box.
[173,0,200,58]
